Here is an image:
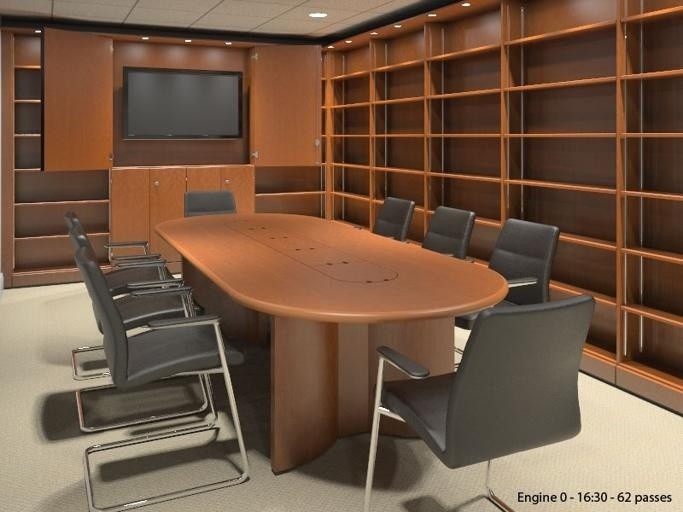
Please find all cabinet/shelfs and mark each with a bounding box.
[320,0,683,410]
[0,26,110,289]
[246,44,323,168]
[186,165,256,214]
[41,28,113,173]
[109,166,186,265]
[258,164,318,219]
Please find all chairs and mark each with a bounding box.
[73,246,248,512]
[185,188,232,212]
[367,196,414,242]
[454,218,559,369]
[361,294,597,512]
[67,227,215,432]
[421,205,475,258]
[64,205,173,382]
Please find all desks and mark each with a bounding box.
[152,213,510,474]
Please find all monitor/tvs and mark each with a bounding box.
[122,65,244,140]
